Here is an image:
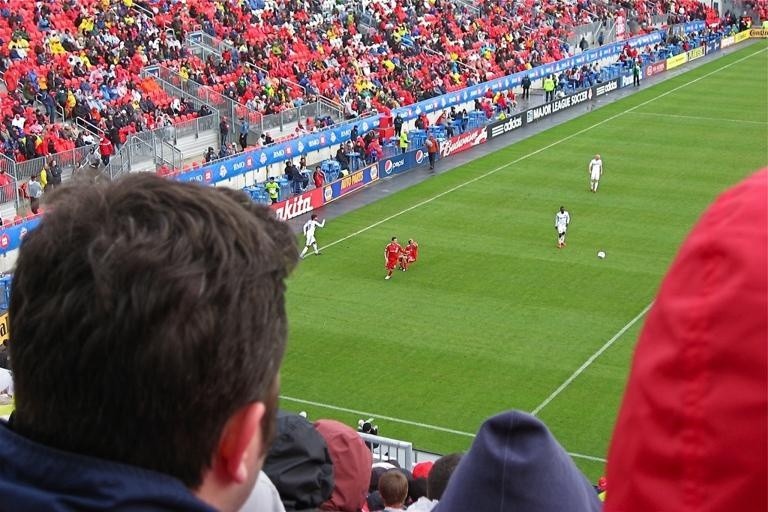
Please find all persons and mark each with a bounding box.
[0,172,299,512]
[384,237,418,280]
[1,339,16,406]
[300,214,326,258]
[602,156,766,511]
[554,206,570,248]
[426,0,767,137]
[237,408,608,512]
[0,0,438,209]
[589,155,603,192]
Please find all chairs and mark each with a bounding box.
[261,1,442,214]
[442,1,717,132]
[0,0,262,307]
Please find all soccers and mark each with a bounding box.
[598,252,606,259]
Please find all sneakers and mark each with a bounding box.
[384,275,391,280]
[561,242,566,246]
[402,267,408,271]
[558,244,561,248]
[591,189,593,192]
[314,252,321,256]
[594,189,596,193]
[299,253,305,260]
[399,267,403,269]
